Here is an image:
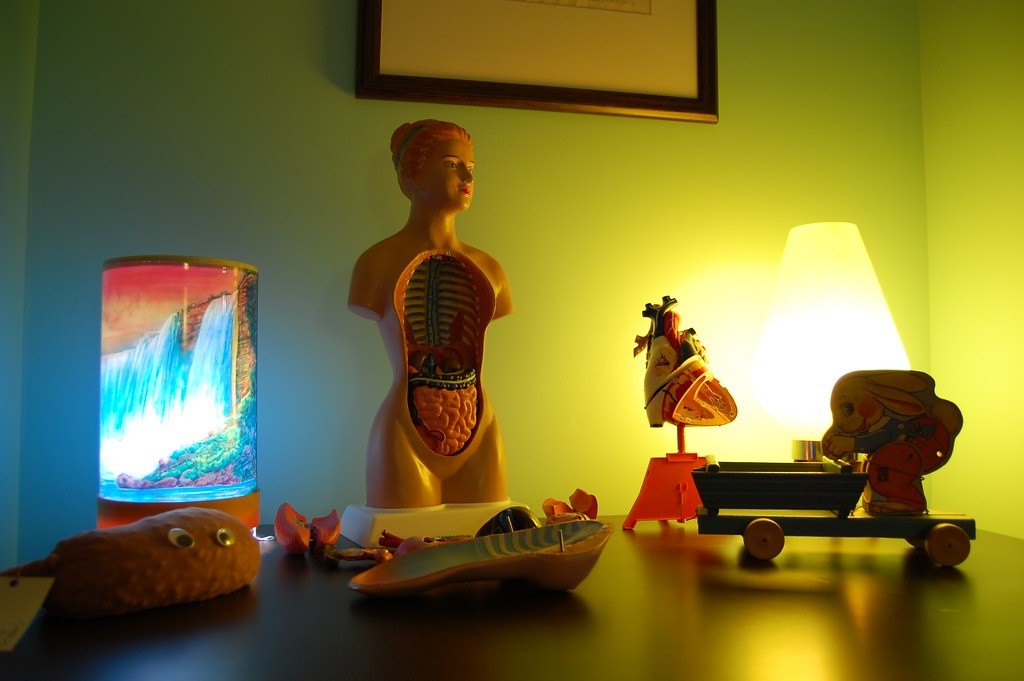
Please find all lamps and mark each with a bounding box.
[97,254,259,531]
[749,220,911,461]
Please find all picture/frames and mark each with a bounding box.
[353,0,721,125]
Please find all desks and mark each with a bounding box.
[0,515,1024,681]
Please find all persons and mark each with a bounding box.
[346,120,512,508]
